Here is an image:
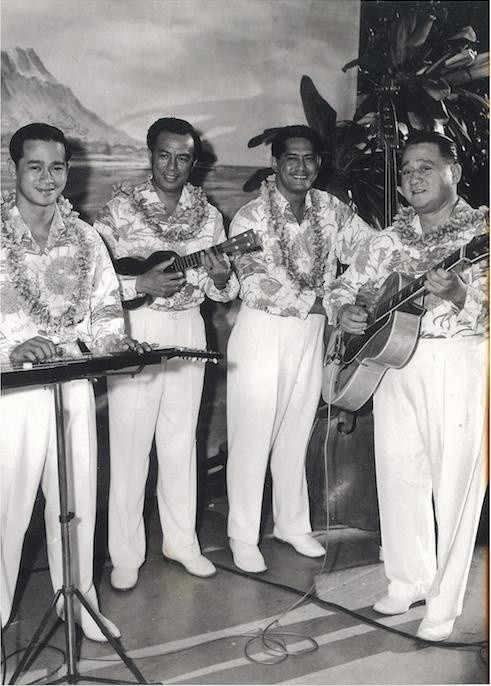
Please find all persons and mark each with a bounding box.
[226,125,380,574]
[1,123,158,643]
[91,117,240,594]
[321,130,489,644]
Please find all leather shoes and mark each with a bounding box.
[229,538,268,573]
[163,552,217,577]
[416,610,456,643]
[272,527,327,558]
[110,566,140,590]
[56,597,122,642]
[372,593,425,615]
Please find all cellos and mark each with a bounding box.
[304,30,407,533]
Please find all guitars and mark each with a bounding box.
[114,228,261,309]
[321,232,489,413]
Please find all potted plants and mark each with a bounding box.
[244,7,488,530]
